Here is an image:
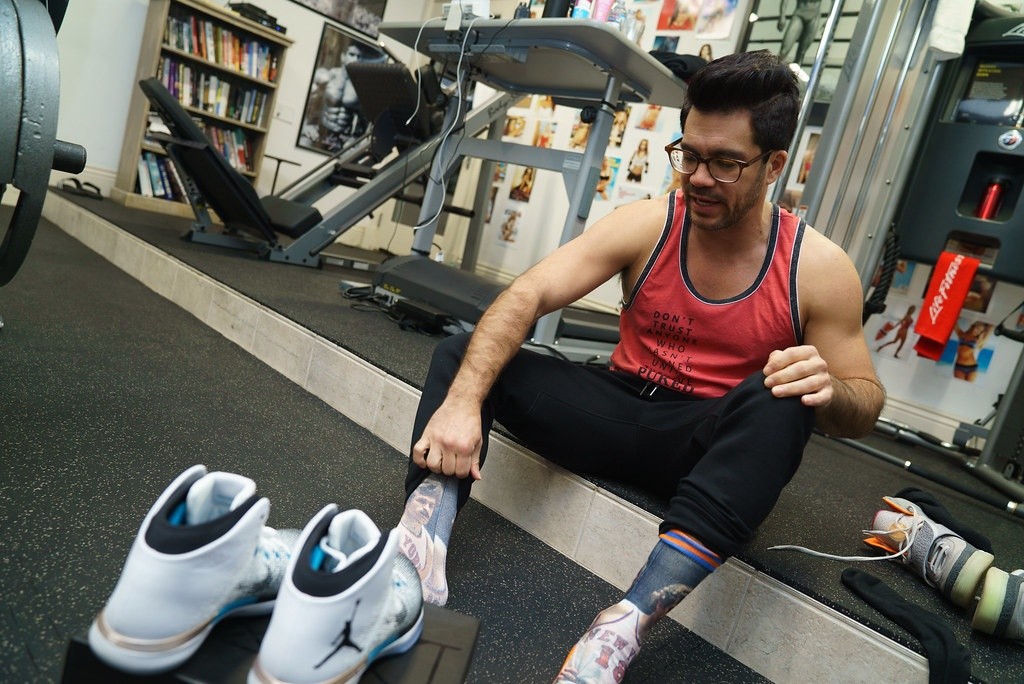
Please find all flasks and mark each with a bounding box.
[975,175,1005,220]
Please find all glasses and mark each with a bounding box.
[664,137,776,183]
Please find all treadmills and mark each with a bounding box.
[371,13,695,367]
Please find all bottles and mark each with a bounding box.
[571,0,590,19]
[605,0,628,32]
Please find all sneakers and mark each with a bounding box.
[89,461,302,674]
[970,568,1024,640]
[862,494,994,606]
[244,502,424,683]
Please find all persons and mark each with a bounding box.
[635,0,725,62]
[302,43,369,164]
[537,123,551,148]
[502,214,516,240]
[640,104,662,132]
[514,167,530,200]
[608,110,627,146]
[877,305,915,358]
[798,135,821,184]
[571,122,590,150]
[897,260,907,273]
[954,317,993,382]
[394,51,885,684]
[627,139,649,182]
[596,158,613,200]
[538,95,554,122]
[494,162,506,180]
[507,117,525,136]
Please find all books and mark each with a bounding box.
[156,16,278,127]
[138,110,253,206]
